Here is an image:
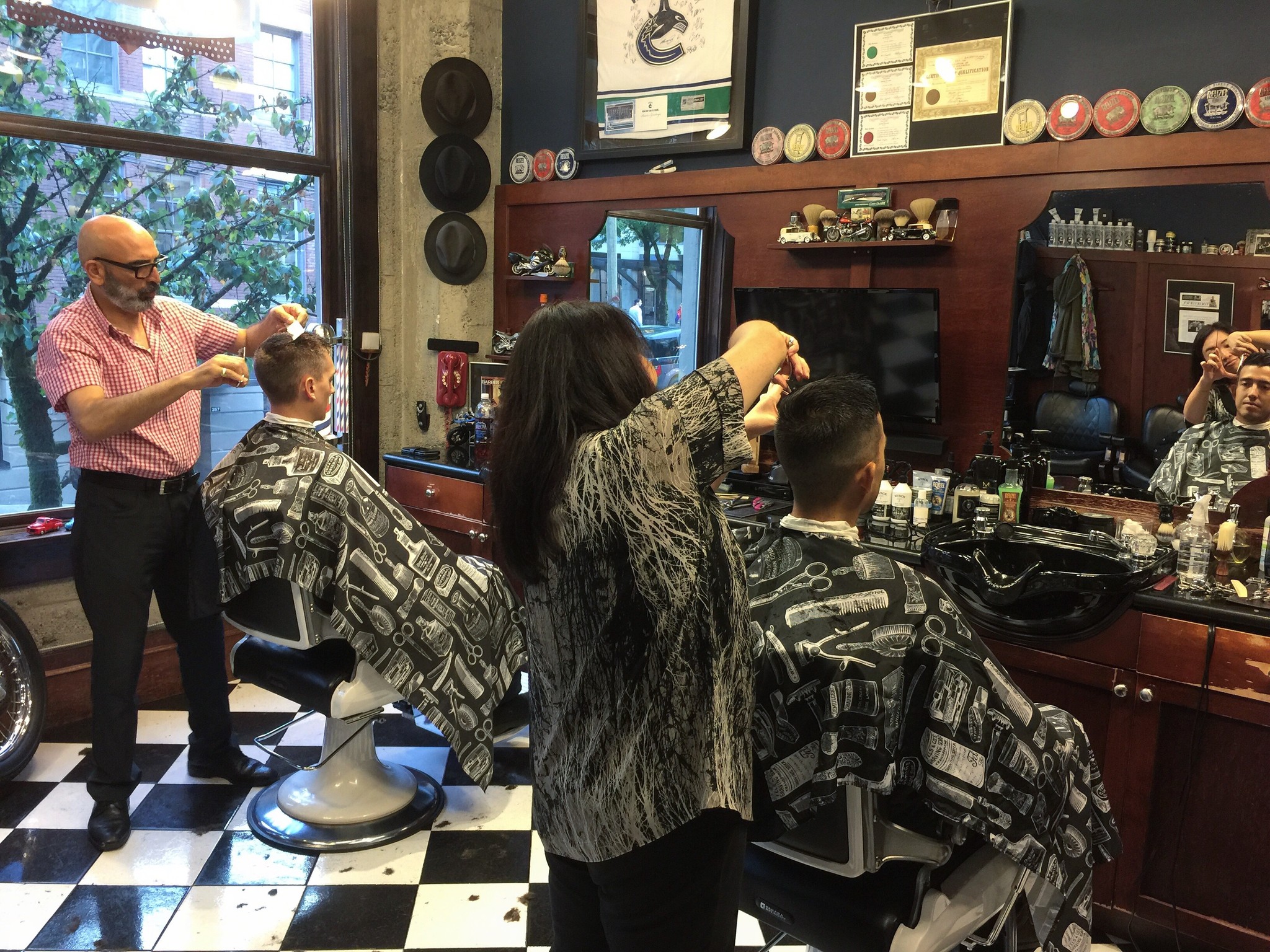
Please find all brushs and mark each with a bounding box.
[360,331,381,386]
[1215,521,1236,576]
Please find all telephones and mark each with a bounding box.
[436,351,468,408]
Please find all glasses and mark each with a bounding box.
[94,254,169,279]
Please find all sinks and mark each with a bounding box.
[923,516,1176,643]
[1068,483,1156,502]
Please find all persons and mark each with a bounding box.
[480,302,811,952]
[628,299,644,328]
[1209,296,1217,307]
[1183,322,1270,430]
[730,376,1122,952]
[1146,353,1270,515]
[198,331,528,795]
[32,214,309,851]
[607,296,620,308]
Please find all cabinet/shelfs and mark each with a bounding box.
[383,235,1270,952]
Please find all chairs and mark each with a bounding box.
[736,639,1038,952]
[202,493,494,852]
[1113,395,1188,492]
[1019,377,1121,482]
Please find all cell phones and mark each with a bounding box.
[401,446,440,456]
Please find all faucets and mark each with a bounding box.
[1087,528,1134,559]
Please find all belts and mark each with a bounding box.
[80,464,201,494]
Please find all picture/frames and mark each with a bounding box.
[574,1,757,160]
[1245,229,1270,257]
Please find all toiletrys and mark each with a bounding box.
[1000,432,1035,523]
[871,429,1001,533]
[1046,461,1055,490]
[997,457,1026,523]
[1176,494,1213,588]
[474,393,495,445]
[1212,503,1253,565]
[1029,429,1051,488]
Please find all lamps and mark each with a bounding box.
[209,60,242,91]
[0,31,42,74]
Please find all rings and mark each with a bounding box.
[221,368,226,377]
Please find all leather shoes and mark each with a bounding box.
[86,795,130,850]
[187,752,278,786]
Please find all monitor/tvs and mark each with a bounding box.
[733,287,943,426]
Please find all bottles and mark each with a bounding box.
[952,457,1023,524]
[912,490,929,528]
[872,473,911,524]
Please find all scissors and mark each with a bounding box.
[1237,352,1247,373]
[236,347,245,389]
[773,337,797,392]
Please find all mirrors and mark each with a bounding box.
[1002,182,1270,513]
[587,206,734,394]
